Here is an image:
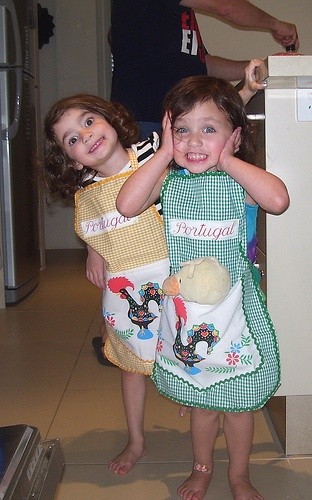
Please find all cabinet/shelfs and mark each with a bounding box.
[234,51,311,457]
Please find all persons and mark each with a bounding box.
[116,74,290,499]
[109,0,301,144]
[39,58,268,476]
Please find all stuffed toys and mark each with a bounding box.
[161,255,231,305]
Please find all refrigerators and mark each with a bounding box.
[0,0,45,305]
